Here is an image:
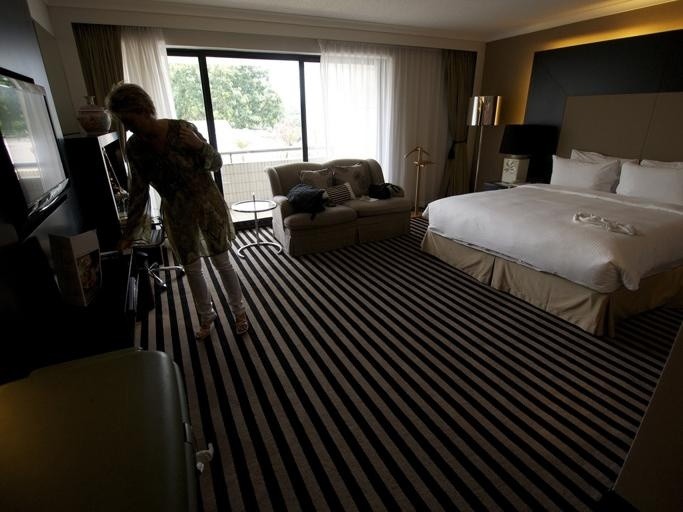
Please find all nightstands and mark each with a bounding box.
[484,180,523,190]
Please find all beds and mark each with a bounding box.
[418,92,683,338]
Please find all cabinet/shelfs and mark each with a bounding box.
[65,130,132,240]
[1,250,136,366]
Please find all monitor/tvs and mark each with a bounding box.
[0,67,71,242]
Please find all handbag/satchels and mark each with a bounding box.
[368,183,390,200]
[286,183,325,213]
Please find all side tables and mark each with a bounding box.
[231,199,283,258]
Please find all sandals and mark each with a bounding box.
[235,311,249,334]
[195,319,212,340]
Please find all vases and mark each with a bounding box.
[75,96,111,133]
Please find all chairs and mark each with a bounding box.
[122,217,184,289]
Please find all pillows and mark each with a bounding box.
[333,160,373,198]
[615,162,683,206]
[639,159,683,171]
[550,155,619,192]
[569,148,639,174]
[297,166,332,189]
[324,182,355,204]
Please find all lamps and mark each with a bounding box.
[498,123,545,184]
[466,96,502,192]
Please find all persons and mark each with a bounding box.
[83,251,101,303]
[107,84,250,340]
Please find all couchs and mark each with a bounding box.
[263,158,410,258]
